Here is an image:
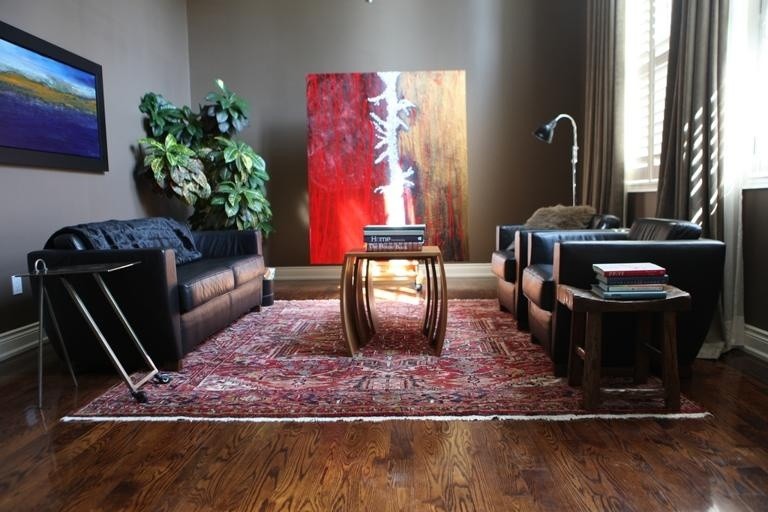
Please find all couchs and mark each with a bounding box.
[26,213,268,375]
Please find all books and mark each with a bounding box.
[363,230,424,235]
[364,235,424,243]
[593,261,666,277]
[595,274,670,286]
[366,242,423,252]
[597,283,666,292]
[590,284,668,301]
[363,222,426,230]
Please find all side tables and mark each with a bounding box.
[22,258,174,407]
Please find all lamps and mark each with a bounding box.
[532,108,580,207]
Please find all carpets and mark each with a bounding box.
[59,294,717,423]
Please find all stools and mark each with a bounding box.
[554,278,697,414]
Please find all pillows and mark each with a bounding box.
[505,204,598,256]
